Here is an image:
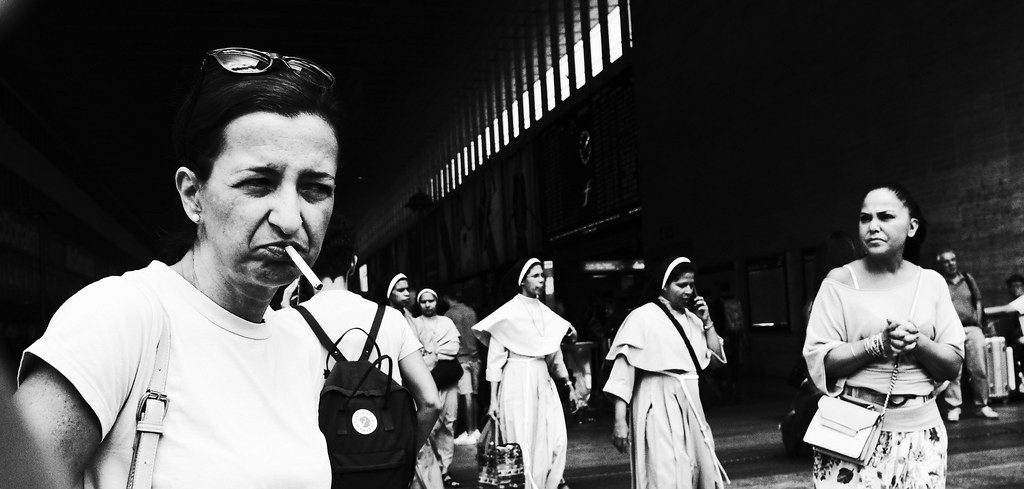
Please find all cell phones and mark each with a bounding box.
[689,285,701,309]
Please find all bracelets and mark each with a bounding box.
[863,333,888,363]
[849,343,861,363]
[564,381,572,386]
[703,321,713,330]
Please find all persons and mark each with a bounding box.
[802,181,967,489]
[934,247,1024,421]
[0,47,593,489]
[602,256,731,489]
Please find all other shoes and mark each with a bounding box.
[975,406,999,418]
[947,408,961,420]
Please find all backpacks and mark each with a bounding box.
[292,302,418,489]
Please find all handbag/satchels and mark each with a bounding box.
[803,394,884,466]
[476,416,525,489]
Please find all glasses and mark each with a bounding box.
[199,47,336,90]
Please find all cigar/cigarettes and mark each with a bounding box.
[285,244,323,291]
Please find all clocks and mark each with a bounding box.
[577,127,593,170]
